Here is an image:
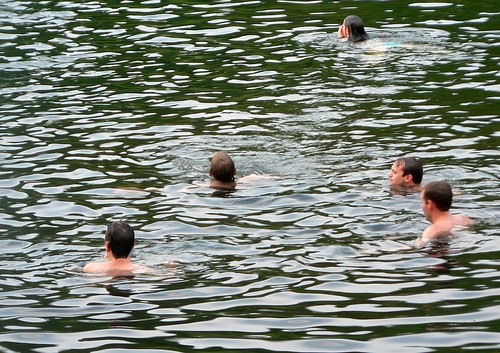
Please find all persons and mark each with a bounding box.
[388,156,425,196]
[418,181,475,248]
[337,15,414,65]
[82,220,179,281]
[190,152,283,192]
